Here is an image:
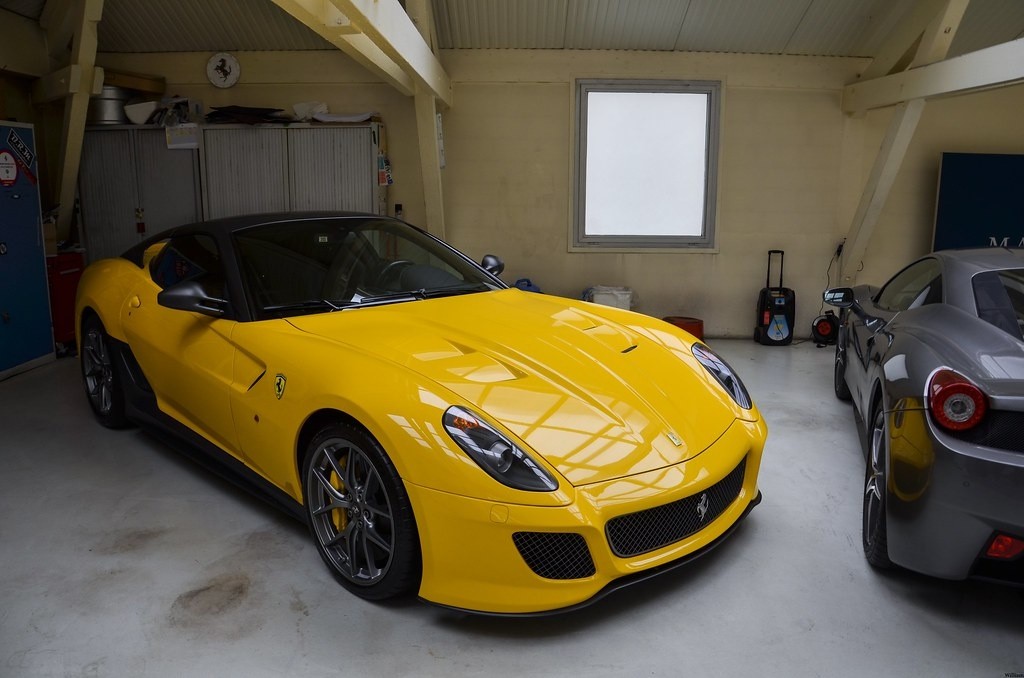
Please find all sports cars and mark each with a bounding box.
[821,246,1024,578]
[72,210,769,620]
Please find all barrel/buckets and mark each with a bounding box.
[663,315,704,344]
[591,286,632,312]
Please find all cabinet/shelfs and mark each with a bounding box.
[78,123,203,267]
[198,110,387,258]
